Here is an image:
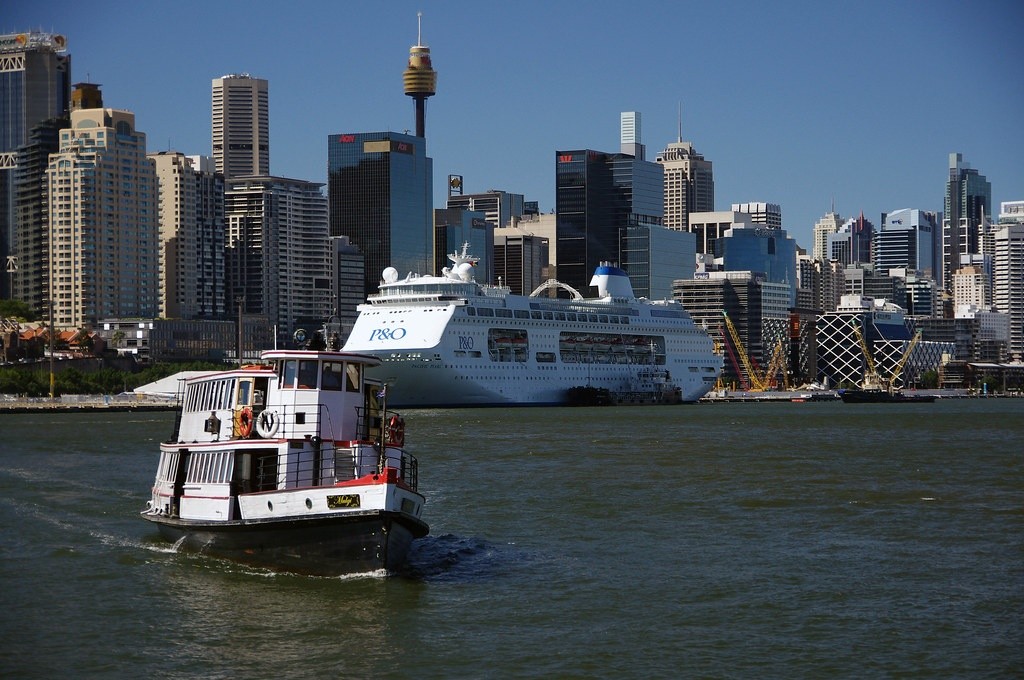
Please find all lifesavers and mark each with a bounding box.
[255,408,280,438]
[238,407,253,439]
[390,415,403,446]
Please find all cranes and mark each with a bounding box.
[718,310,790,394]
[888,330,924,387]
[850,319,884,391]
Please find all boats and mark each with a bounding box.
[593,338,613,351]
[512,337,528,348]
[141,324,430,575]
[575,338,594,350]
[633,339,651,354]
[558,339,576,348]
[496,338,513,348]
[610,337,631,352]
[835,388,937,403]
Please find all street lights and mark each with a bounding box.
[42,299,58,402]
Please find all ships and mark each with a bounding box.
[340,239,725,406]
